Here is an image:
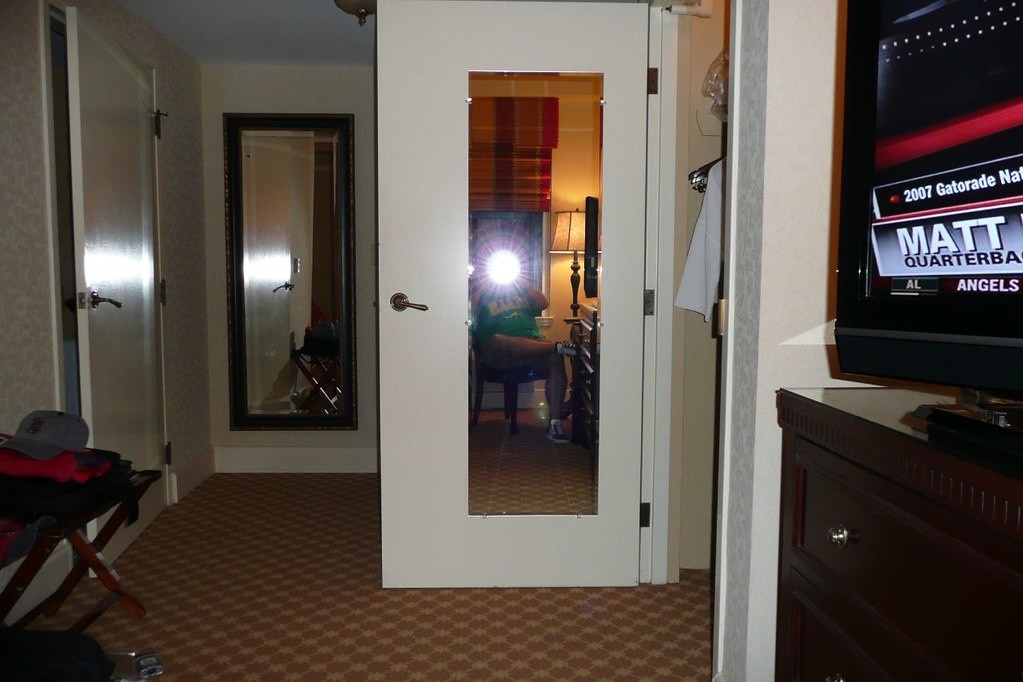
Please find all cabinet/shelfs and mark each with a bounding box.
[776,387,1023,682]
[564,303,600,452]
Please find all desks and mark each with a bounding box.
[0,470,162,630]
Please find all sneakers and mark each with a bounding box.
[554,340,580,358]
[545,418,570,444]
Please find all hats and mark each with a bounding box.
[0,408,89,460]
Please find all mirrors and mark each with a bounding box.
[467,70,604,516]
[222,113,359,431]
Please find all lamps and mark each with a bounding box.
[548,208,586,317]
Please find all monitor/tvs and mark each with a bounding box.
[832,1,1023,460]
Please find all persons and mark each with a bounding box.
[470,255,571,444]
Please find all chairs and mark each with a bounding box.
[471,331,547,437]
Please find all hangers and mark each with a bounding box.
[689,157,724,193]
